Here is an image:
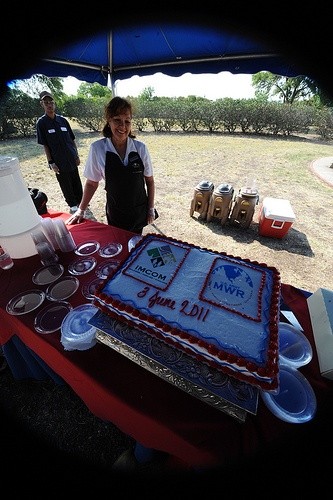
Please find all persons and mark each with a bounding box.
[36,91,85,212]
[65,97,154,235]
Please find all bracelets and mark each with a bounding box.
[48,160,54,164]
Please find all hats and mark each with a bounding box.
[40,91,54,100]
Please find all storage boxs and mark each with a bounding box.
[258,197,296,240]
[306,287,333,380]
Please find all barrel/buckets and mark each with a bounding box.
[0,155,42,259]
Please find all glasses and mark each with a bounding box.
[42,101,55,104]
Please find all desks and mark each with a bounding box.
[0,208,333,500]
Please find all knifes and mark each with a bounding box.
[279,292,304,331]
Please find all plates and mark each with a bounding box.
[260,322,318,424]
[6,289,45,316]
[33,301,100,342]
[97,240,123,258]
[74,240,101,256]
[94,258,121,279]
[45,276,79,301]
[68,256,97,275]
[81,274,101,301]
[32,263,64,285]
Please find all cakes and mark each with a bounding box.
[92,233,282,391]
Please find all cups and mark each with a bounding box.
[0,245,14,270]
[30,217,76,266]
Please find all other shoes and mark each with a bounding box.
[83,209,97,221]
[69,209,78,214]
